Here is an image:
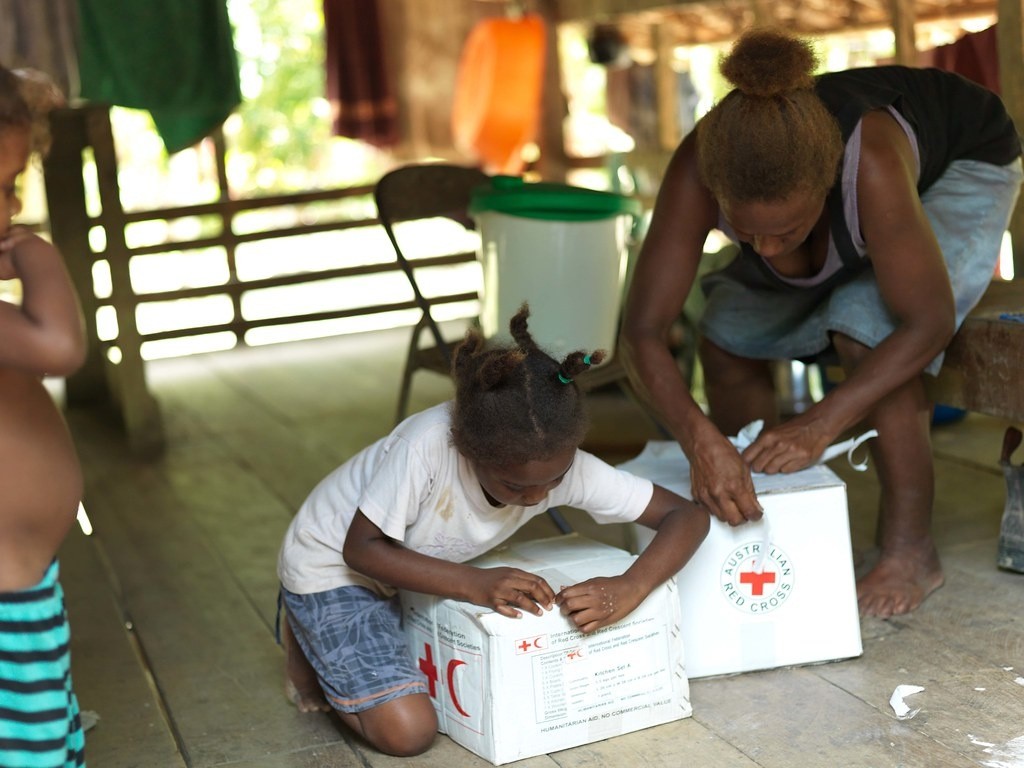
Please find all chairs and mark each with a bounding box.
[373,161,674,536]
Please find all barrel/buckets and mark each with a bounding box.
[468,175,643,371]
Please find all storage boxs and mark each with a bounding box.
[397,532,693,766]
[615,449,864,679]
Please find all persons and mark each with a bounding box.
[276,304,711,757]
[616,30,1024,619]
[0,68,88,768]
